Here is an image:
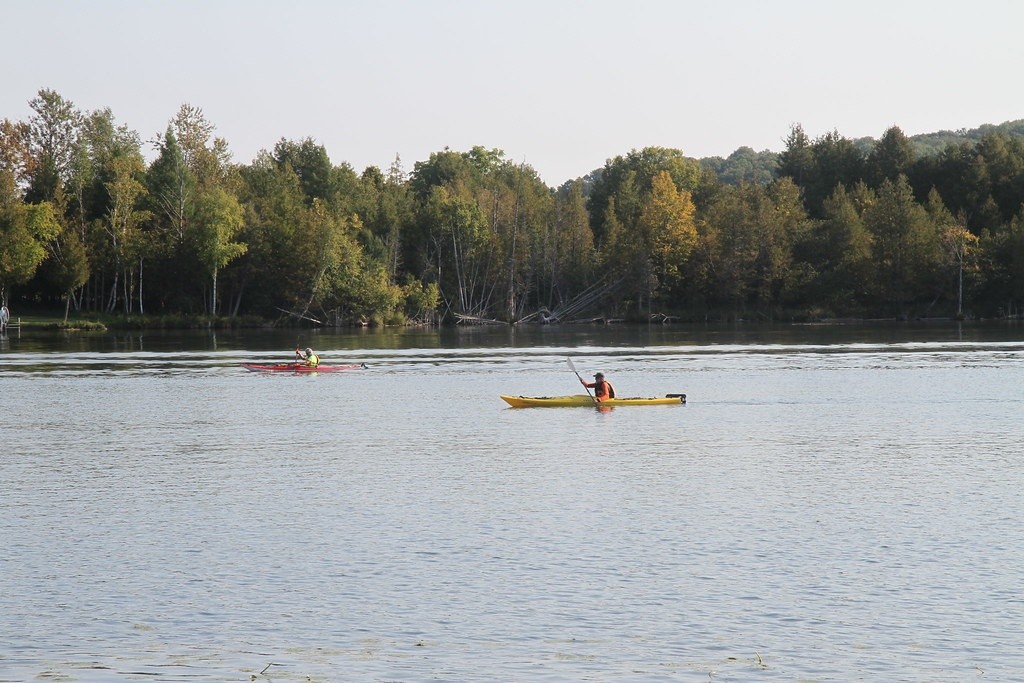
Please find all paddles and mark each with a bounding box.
[293,334,302,377]
[565,356,599,407]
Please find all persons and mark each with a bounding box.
[582,373,614,411]
[299,347,319,368]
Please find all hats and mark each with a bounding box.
[593,372,604,378]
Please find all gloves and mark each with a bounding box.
[296,350,301,355]
[295,362,300,366]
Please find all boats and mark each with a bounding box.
[500,393,687,408]
[239,360,366,371]
